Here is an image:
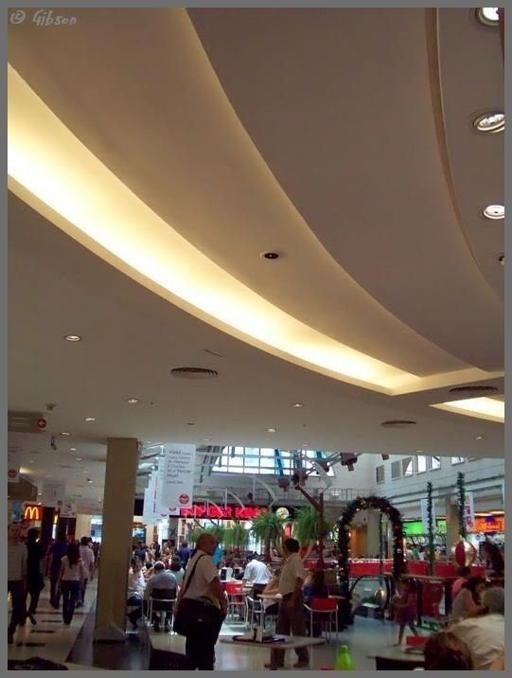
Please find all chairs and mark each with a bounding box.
[223,580,338,646]
[128,587,176,632]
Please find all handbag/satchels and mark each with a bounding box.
[173,599,221,641]
[48,583,85,626]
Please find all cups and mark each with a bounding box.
[265,620,276,636]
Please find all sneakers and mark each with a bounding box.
[265,660,310,670]
[130,618,171,634]
[8,630,15,645]
[19,612,37,626]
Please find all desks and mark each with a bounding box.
[365,644,425,670]
[220,634,327,671]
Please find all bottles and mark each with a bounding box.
[334,645,354,670]
